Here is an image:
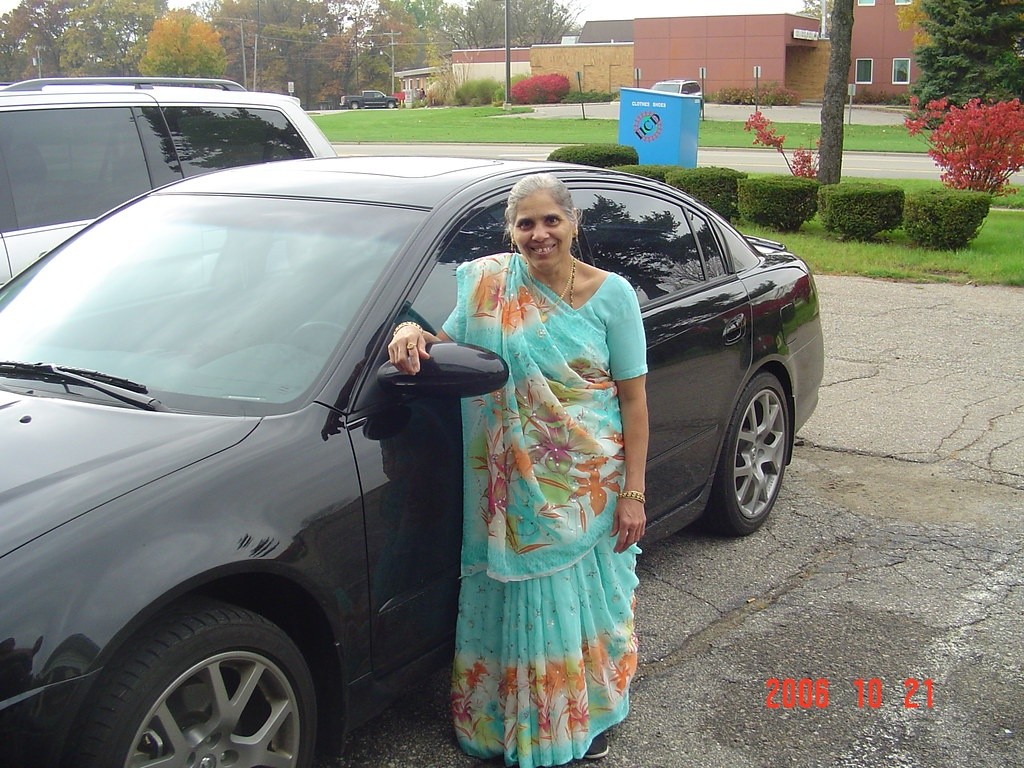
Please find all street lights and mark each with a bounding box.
[380,49,394,96]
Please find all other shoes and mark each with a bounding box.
[584,732,608,759]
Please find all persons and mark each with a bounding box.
[387,174,650,768]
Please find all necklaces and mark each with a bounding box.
[526,256,573,322]
[570,258,576,309]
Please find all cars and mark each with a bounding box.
[0,155,825,768]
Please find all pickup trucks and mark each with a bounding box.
[339,91,398,109]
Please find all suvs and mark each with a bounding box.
[0,78,338,285]
[651,80,703,110]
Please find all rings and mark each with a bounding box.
[406,342,416,349]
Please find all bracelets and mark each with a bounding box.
[616,490,646,504]
[393,322,422,336]
